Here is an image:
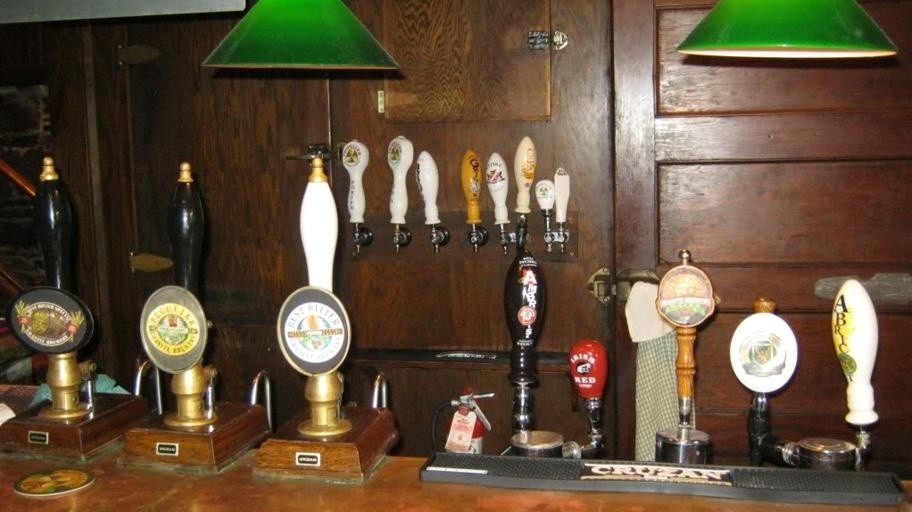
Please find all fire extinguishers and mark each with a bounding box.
[430,386,496,455]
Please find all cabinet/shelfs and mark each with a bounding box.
[96,14,309,407]
[330,2,906,462]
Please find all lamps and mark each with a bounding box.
[676,3,899,62]
[201,0,401,75]
